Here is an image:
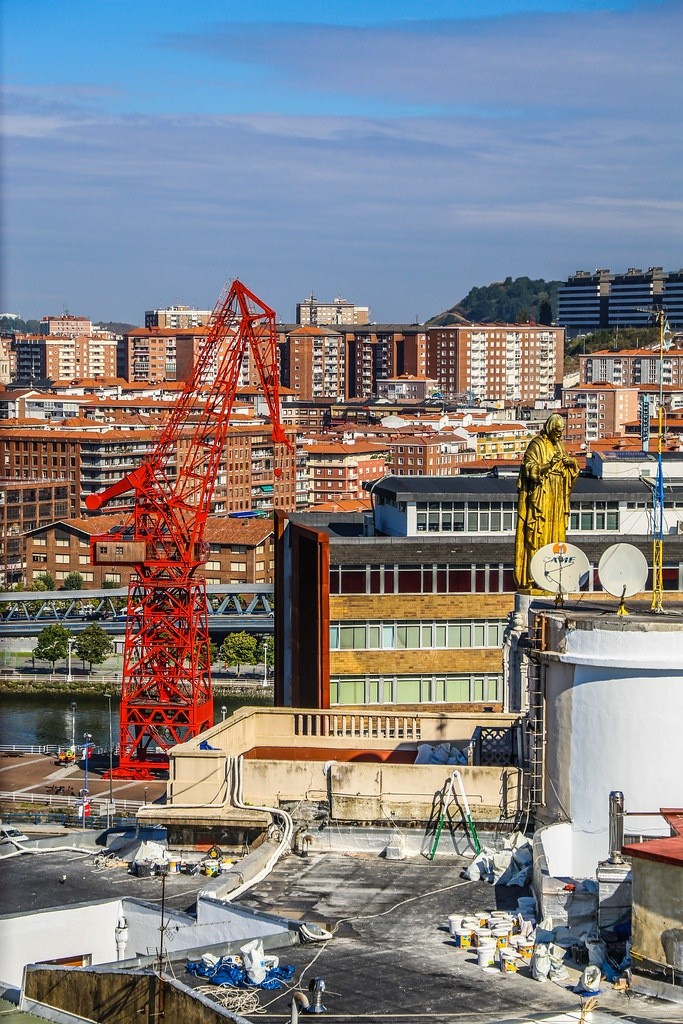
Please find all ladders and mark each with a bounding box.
[429,770,481,860]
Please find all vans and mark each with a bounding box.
[113,605,144,622]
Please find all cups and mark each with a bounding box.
[204,859,219,877]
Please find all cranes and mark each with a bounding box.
[84,274,296,781]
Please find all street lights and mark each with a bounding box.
[103,693,112,803]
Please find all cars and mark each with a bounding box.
[82,611,109,622]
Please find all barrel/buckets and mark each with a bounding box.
[220,863,234,875]
[135,858,152,877]
[184,861,200,876]
[168,861,176,872]
[447,896,580,975]
[176,861,181,872]
[155,863,168,876]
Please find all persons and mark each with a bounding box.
[514,414,580,587]
